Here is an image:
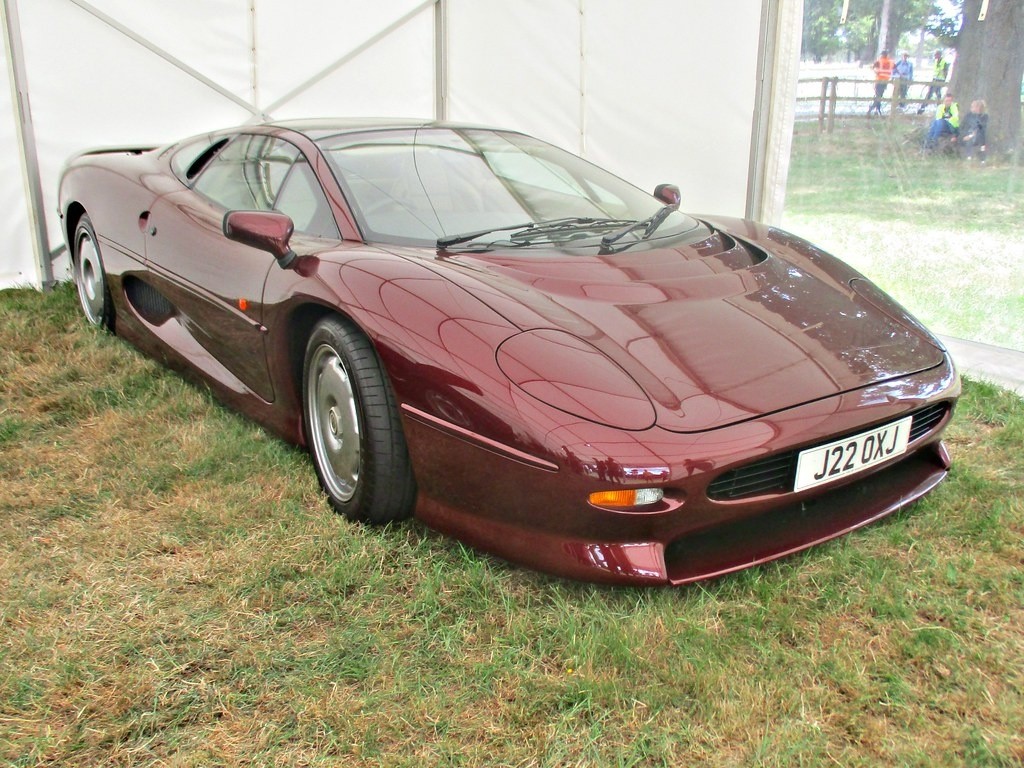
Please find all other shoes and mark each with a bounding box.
[868,105,872,114]
[916,107,925,115]
[875,112,882,115]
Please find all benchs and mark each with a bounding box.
[225,180,424,218]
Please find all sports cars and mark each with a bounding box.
[56,118,964,589]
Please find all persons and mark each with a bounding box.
[925,93,960,146]
[867,48,948,116]
[952,100,988,165]
